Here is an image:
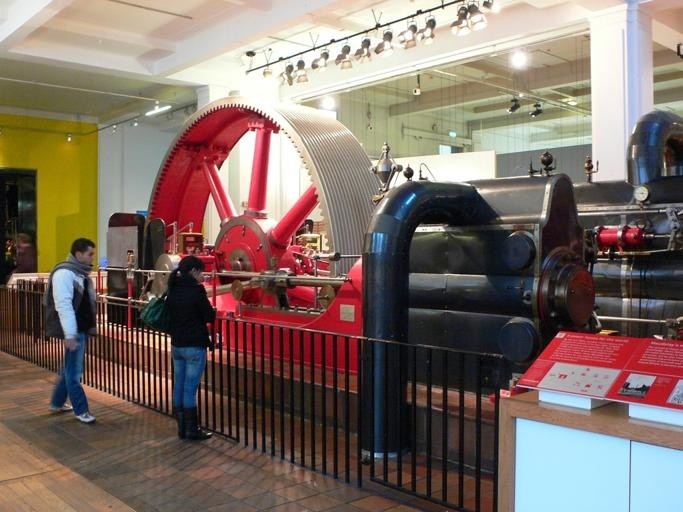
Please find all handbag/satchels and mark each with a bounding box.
[139,289,168,332]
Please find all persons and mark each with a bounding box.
[165,256,217,440]
[43,236,99,423]
[5,231,35,277]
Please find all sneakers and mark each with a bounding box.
[73,411,95,424]
[47,403,72,413]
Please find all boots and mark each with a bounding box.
[182,405,213,440]
[173,405,184,440]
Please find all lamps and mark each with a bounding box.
[237,0,496,98]
[505,91,546,122]
[62,100,196,142]
